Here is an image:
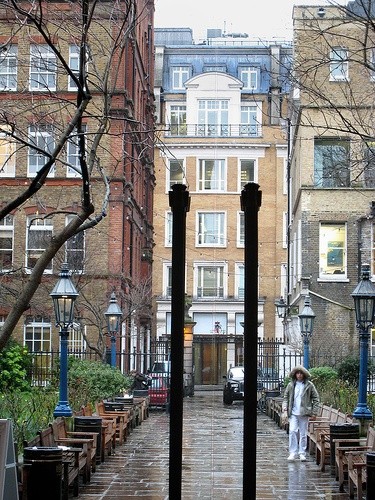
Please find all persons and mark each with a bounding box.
[130,370,147,390]
[281,364,319,462]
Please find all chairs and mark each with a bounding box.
[265,390,375,500]
[25,390,150,500]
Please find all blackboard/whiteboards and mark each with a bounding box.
[0,418,19,500]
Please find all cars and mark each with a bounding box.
[146,360,170,411]
[221,366,268,405]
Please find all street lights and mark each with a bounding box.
[349,262,375,420]
[48,262,81,418]
[296,295,317,371]
[103,292,123,367]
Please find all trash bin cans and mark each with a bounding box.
[115,397,133,403]
[133,390,148,397]
[73,417,102,465]
[329,424,360,478]
[265,391,280,399]
[22,447,62,500]
[366,452,375,500]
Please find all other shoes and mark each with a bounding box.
[288,454,295,461]
[299,454,306,462]
